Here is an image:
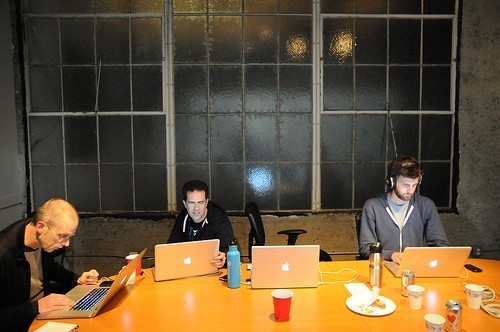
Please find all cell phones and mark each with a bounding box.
[465,264,482,272]
[99,280,114,287]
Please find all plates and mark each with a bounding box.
[346,294,396,316]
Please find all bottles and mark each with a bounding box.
[401,270,415,296]
[227,243,240,289]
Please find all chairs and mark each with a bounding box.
[245,202,332,261]
[353,208,369,259]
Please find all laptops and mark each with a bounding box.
[36,248,147,319]
[251,244,320,288]
[384,246,472,278]
[152,239,219,281]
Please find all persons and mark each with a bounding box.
[0,198,99,332]
[359,158,450,265]
[167,180,234,268]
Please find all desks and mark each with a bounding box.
[25,257,500,332]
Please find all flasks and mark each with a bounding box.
[369,243,383,287]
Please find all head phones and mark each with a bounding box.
[385,164,395,189]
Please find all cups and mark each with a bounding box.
[424,314,446,332]
[122,254,142,284]
[272,289,294,321]
[465,283,484,309]
[407,285,425,310]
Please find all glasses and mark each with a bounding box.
[46,223,72,242]
[186,201,206,208]
